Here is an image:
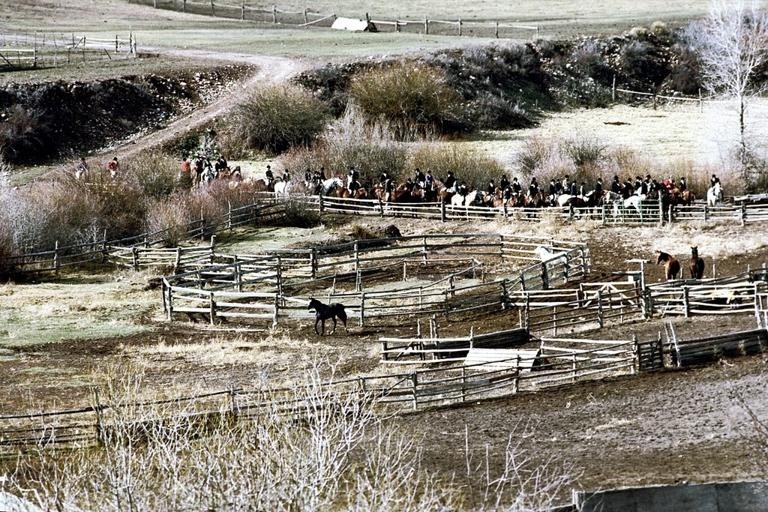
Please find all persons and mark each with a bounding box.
[347,165,359,198]
[305,165,326,194]
[77,156,90,182]
[180,155,230,180]
[109,156,121,183]
[380,169,393,185]
[710,174,721,187]
[282,168,290,184]
[404,168,690,222]
[266,165,275,186]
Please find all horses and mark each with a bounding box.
[306,297,348,336]
[688,246,705,278]
[655,250,680,281]
[706,182,724,206]
[266,176,697,221]
[177,167,267,191]
